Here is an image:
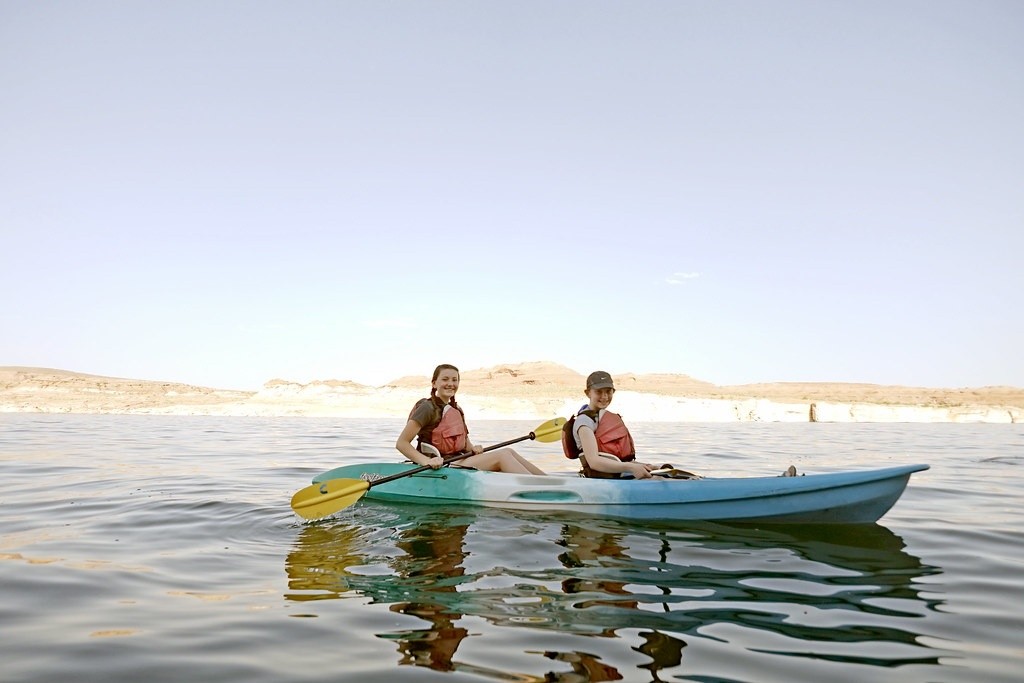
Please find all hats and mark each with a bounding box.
[586,370,616,390]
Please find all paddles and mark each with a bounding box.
[650,468,696,478]
[291,417,568,520]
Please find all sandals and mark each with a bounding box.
[778,465,805,477]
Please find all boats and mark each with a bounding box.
[311,462,932,524]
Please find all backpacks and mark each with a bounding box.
[595,409,636,462]
[561,412,584,459]
[432,404,466,453]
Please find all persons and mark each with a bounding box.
[572,370,800,479]
[395,363,552,476]
